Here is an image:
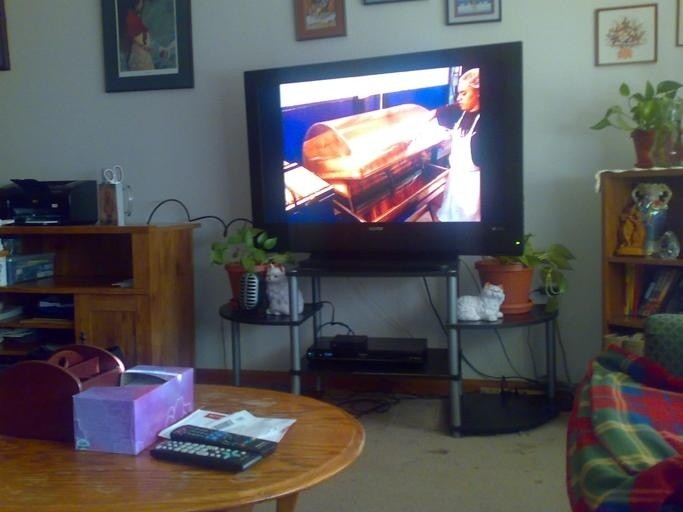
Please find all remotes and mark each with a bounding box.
[149,439,262,472]
[171,423,277,455]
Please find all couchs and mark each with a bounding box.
[561,311,683,512]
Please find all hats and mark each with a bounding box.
[125,12,149,36]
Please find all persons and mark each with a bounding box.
[119,1,168,70]
[417,67,481,224]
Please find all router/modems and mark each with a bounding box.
[331,334,367,349]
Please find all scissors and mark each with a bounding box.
[104,165,123,183]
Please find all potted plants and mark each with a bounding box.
[587,78,682,167]
[209,222,293,308]
[474,232,579,318]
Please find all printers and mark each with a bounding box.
[0,180,99,226]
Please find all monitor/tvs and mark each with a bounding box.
[243,42,524,275]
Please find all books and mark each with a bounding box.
[5,253,58,261]
[13,263,58,271]
[15,271,57,282]
[160,408,298,445]
[0,300,27,320]
[622,262,683,318]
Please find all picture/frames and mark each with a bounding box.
[594,2,657,67]
[294,0,346,41]
[100,0,196,93]
[445,0,502,25]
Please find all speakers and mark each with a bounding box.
[239,273,264,312]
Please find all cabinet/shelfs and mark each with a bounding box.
[216,257,572,438]
[0,220,203,382]
[597,167,683,356]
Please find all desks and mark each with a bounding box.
[1,377,367,511]
